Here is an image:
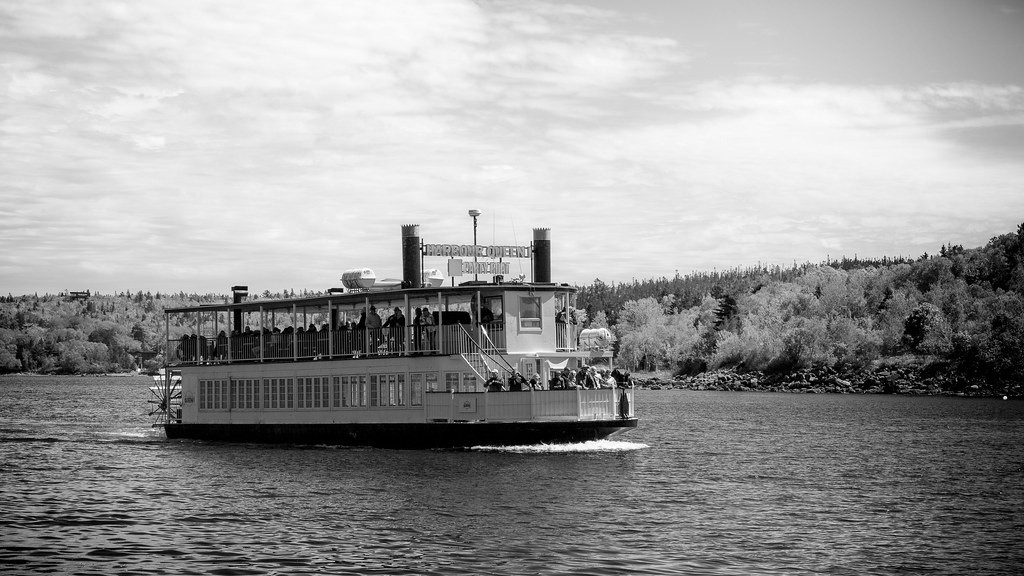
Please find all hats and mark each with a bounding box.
[556,371,561,376]
[564,367,571,371]
[422,308,428,312]
[370,306,376,309]
[492,369,498,373]
[581,364,589,369]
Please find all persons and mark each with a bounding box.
[529,373,543,390]
[174,306,435,364]
[483,369,503,392]
[549,365,632,391]
[476,302,494,330]
[508,370,527,392]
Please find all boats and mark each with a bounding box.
[146,211,639,449]
